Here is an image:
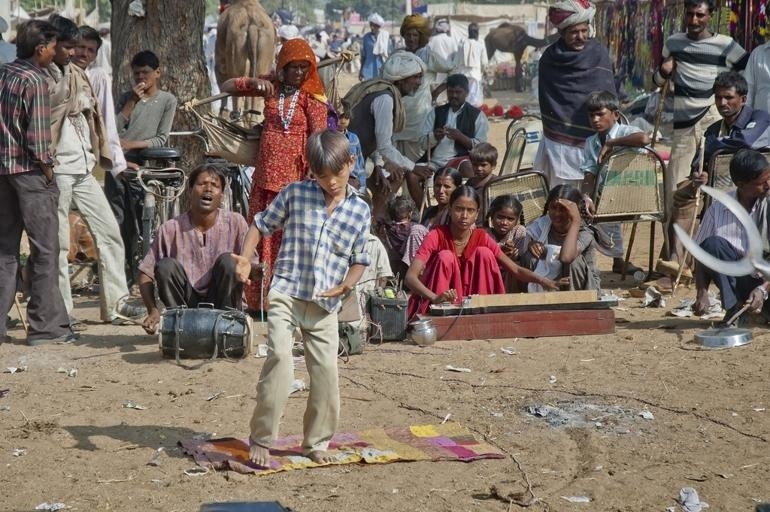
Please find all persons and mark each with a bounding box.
[71,25,139,177]
[46,14,147,332]
[0,20,80,346]
[0,16,17,65]
[228,130,371,468]
[104,50,178,286]
[96,27,110,72]
[137,164,258,334]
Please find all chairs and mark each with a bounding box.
[483,171,549,231]
[498,127,526,177]
[507,115,542,171]
[703,150,770,215]
[590,147,668,280]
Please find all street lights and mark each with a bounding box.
[331,6,345,33]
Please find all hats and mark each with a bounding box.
[382,49,429,83]
[400,13,433,48]
[435,17,451,34]
[547,0,599,28]
[367,11,384,28]
[277,23,299,41]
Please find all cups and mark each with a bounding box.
[408,319,437,346]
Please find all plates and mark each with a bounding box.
[694,328,753,349]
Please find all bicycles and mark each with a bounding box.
[127,117,207,268]
[215,111,262,216]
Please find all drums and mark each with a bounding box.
[159,308,254,359]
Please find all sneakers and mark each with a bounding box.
[26,332,81,346]
[64,316,88,331]
[110,301,149,325]
